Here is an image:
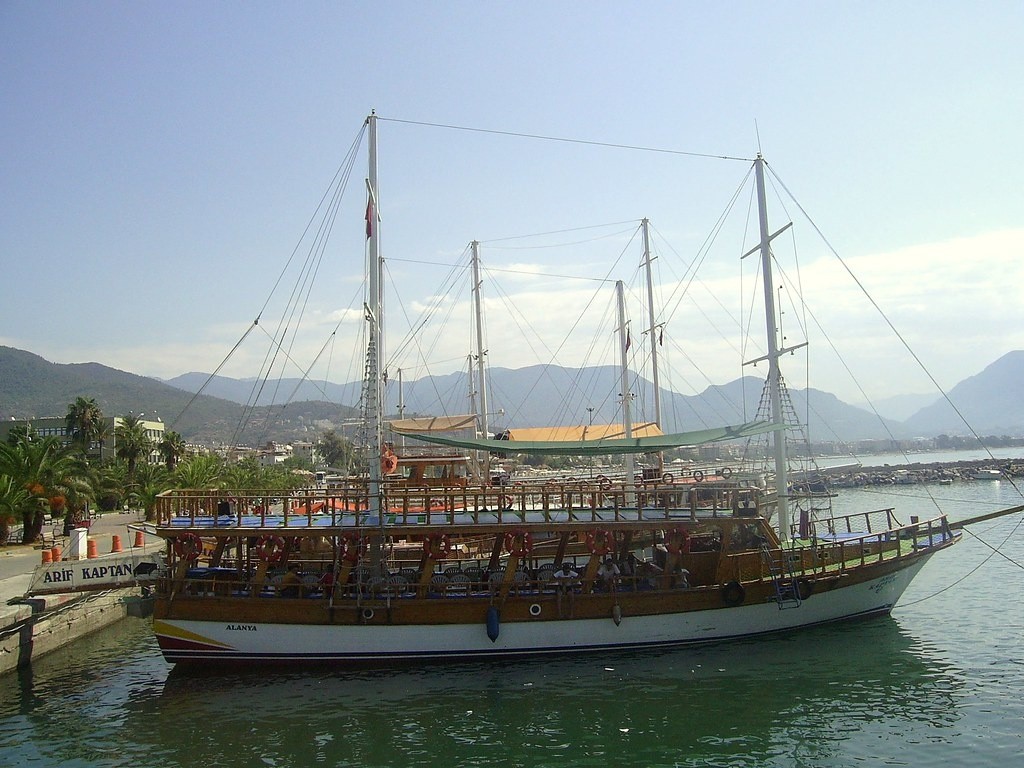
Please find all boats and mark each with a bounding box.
[786,458,1024,492]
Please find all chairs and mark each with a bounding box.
[301,562,573,594]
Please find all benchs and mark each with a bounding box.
[9,528,24,544]
[43,515,59,525]
[121,505,135,514]
[88,509,103,518]
[40,532,65,548]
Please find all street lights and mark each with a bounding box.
[23,416,36,443]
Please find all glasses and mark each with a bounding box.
[564,571,570,573]
[608,564,614,567]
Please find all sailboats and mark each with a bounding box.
[275,216,840,521]
[6,106,1024,669]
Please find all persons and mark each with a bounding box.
[319,564,335,597]
[281,566,304,598]
[597,559,620,586]
[554,565,579,592]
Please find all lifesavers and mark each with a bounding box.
[497,495,513,509]
[256,534,284,562]
[429,499,443,507]
[567,477,589,490]
[423,531,452,559]
[548,479,564,492]
[175,532,202,560]
[513,482,525,493]
[662,472,674,483]
[418,483,493,491]
[681,467,703,482]
[634,476,643,488]
[721,467,732,479]
[340,532,366,562]
[792,577,813,600]
[586,528,614,555]
[722,581,746,605]
[596,474,612,490]
[505,528,532,557]
[664,528,691,555]
